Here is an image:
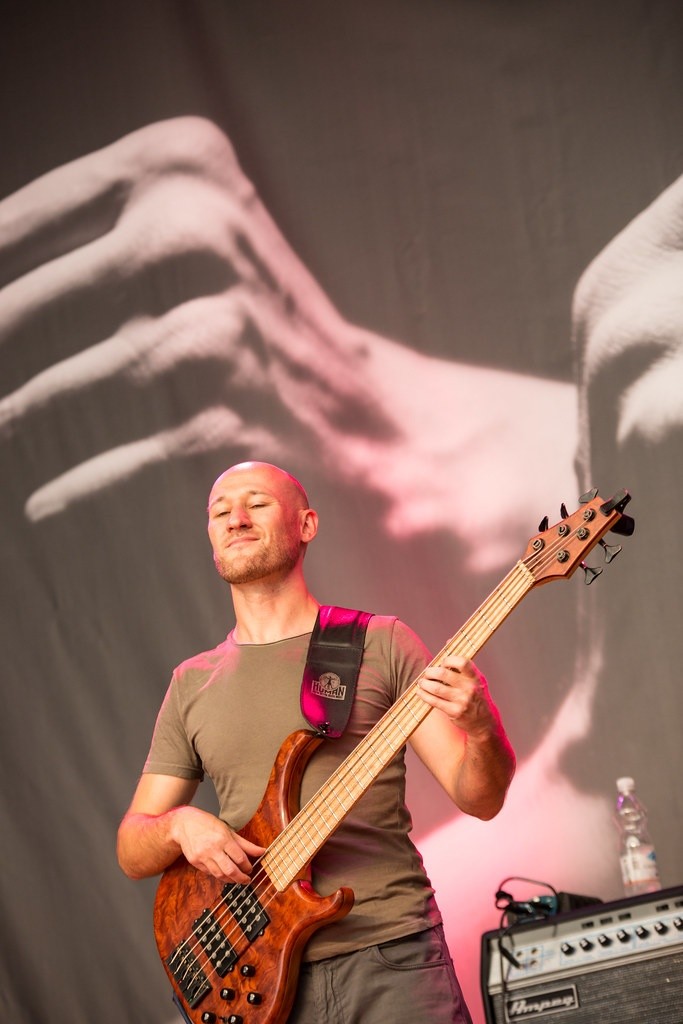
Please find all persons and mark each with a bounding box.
[113,460,518,1024]
[0,115,680,1019]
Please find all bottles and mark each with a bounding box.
[614,777,663,899]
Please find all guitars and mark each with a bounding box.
[153,484,639,1024]
